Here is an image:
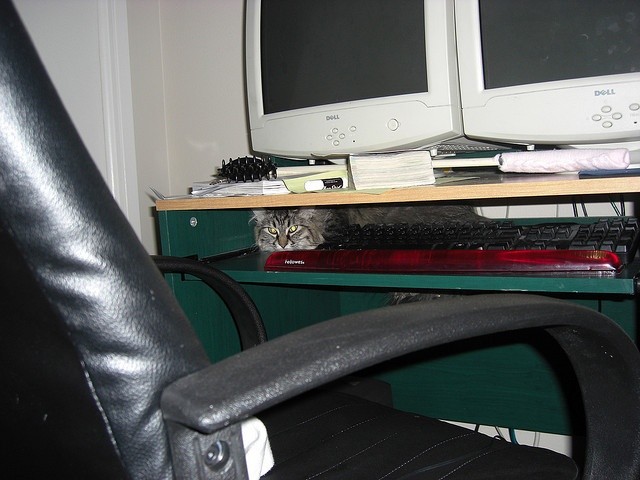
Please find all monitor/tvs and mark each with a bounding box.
[238,0,465,166]
[454,0,639,174]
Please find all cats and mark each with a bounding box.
[246,204,495,253]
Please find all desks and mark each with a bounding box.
[153,171,636,437]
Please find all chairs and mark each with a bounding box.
[0,0,636,480]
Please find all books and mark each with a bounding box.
[192,171,348,196]
[348,149,436,190]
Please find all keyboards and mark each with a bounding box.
[431,142,549,170]
[263,214,639,277]
